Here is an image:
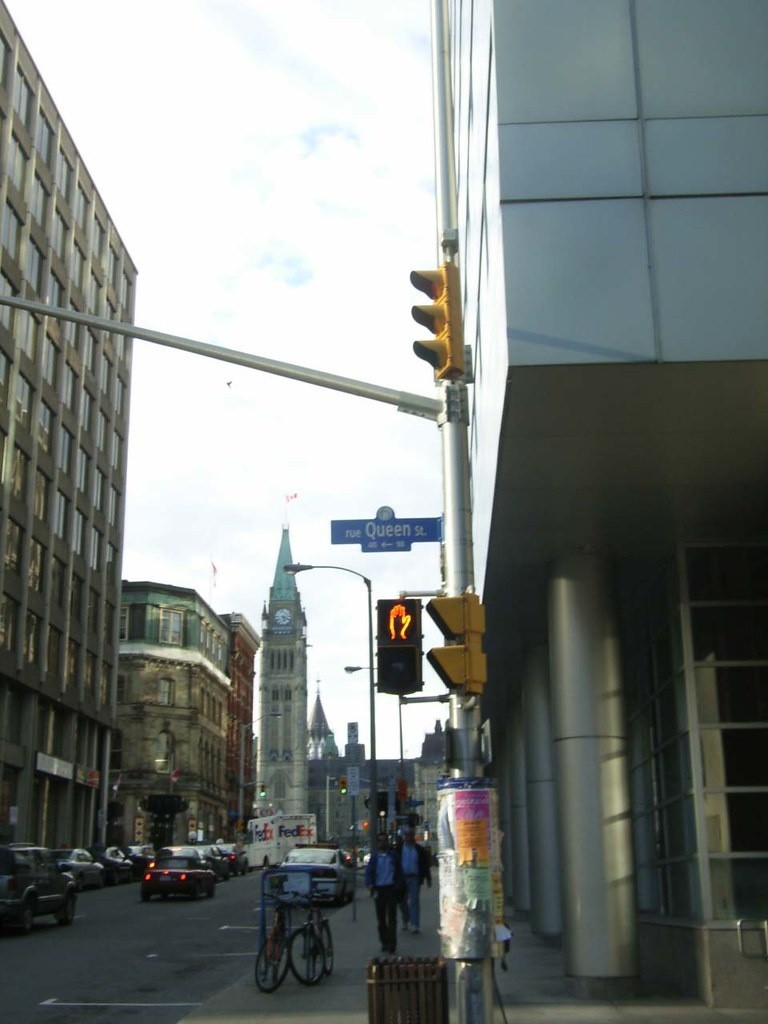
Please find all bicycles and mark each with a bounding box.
[256,886,335,992]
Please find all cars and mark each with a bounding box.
[342,851,354,866]
[276,845,355,905]
[121,846,156,873]
[87,845,136,886]
[140,855,217,901]
[50,848,106,891]
[164,844,249,882]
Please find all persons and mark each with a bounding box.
[396,827,432,933]
[365,834,406,951]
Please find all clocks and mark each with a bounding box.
[275,609,292,625]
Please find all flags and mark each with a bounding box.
[112,774,121,791]
[172,769,179,782]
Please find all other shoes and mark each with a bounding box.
[412,925,419,934]
[382,944,387,951]
[400,921,408,930]
[388,944,396,951]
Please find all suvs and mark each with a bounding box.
[0,842,78,938]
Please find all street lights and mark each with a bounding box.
[237,712,282,841]
[286,562,376,856]
[344,666,405,813]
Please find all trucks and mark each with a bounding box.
[241,813,317,870]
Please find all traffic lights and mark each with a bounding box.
[424,593,486,696]
[362,819,368,831]
[338,777,348,796]
[260,784,266,797]
[376,599,425,695]
[410,264,463,381]
[377,790,389,818]
